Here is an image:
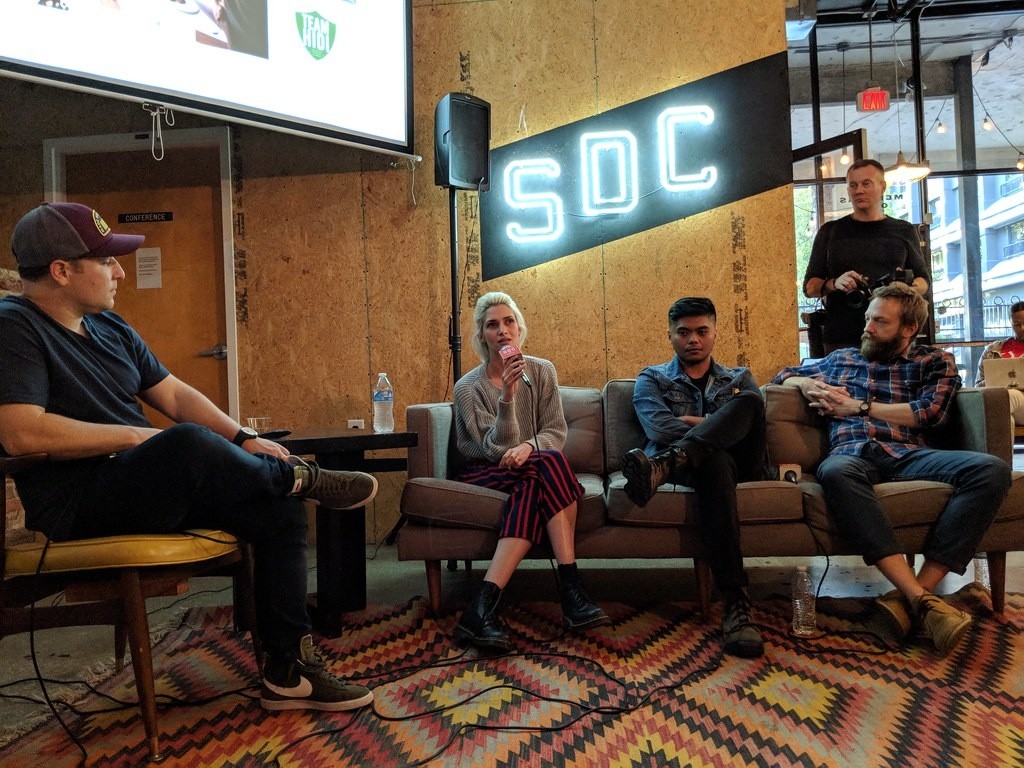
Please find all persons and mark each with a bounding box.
[0,200,378,711]
[620,295,771,659]
[803,159,931,357]
[453,292,611,652]
[974,302,1024,456]
[771,281,1012,652]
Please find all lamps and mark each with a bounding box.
[881,22,930,186]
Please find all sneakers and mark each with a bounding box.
[876,587,920,642]
[907,584,975,655]
[260,632,375,711]
[285,454,378,512]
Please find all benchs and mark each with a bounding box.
[395,379,1024,617]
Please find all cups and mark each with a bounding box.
[943,335,951,340]
[246,418,269,436]
[935,335,942,340]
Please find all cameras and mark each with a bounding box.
[846,269,916,312]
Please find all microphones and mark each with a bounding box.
[498,345,532,388]
[778,463,801,484]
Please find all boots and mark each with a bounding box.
[555,558,613,634]
[451,579,516,657]
[719,586,767,658]
[621,445,688,506]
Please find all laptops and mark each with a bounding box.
[982,358,1024,390]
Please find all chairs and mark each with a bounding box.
[0,450,266,765]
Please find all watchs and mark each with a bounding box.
[232,426,259,447]
[860,401,871,415]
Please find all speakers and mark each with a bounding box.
[434,92,492,192]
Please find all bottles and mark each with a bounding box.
[974,552,991,590]
[793,566,816,635]
[373,373,394,432]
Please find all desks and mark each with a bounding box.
[934,336,1009,348]
[270,427,419,614]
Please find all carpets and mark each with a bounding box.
[0,580,1024,768]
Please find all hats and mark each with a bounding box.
[10,200,146,269]
